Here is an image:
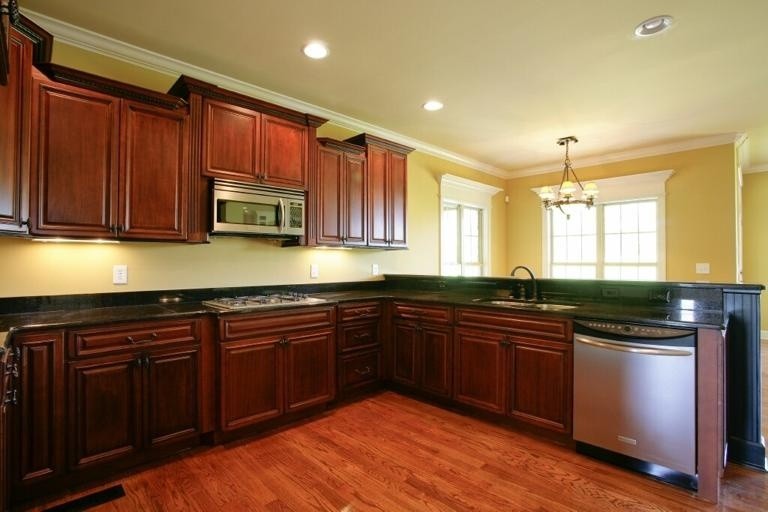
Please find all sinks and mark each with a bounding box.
[478,298,584,311]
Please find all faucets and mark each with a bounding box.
[510,265,539,301]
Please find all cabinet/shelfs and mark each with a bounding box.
[0,0,571,512]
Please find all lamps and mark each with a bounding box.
[531,137,598,224]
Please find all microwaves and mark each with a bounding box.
[208,177,307,238]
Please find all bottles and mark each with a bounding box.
[509,283,527,299]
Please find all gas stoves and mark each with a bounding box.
[201,291,331,313]
